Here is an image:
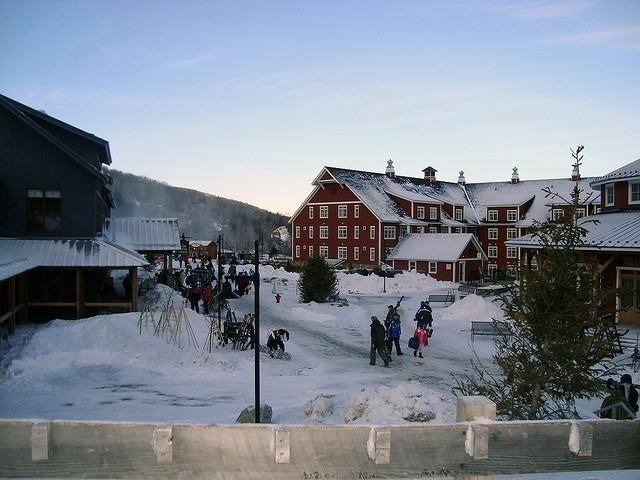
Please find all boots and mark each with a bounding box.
[414,351,423,358]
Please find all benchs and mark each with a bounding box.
[213,321,254,350]
[426,295,455,304]
[471,321,515,342]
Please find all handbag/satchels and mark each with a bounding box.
[409,336,419,349]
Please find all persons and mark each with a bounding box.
[413,321,429,358]
[617,374,640,417]
[123,274,129,295]
[266,328,289,359]
[414,301,432,335]
[383,305,398,331]
[629,345,640,372]
[368,316,390,368]
[599,379,632,419]
[425,301,432,327]
[386,313,405,356]
[377,320,392,362]
[178,247,256,317]
